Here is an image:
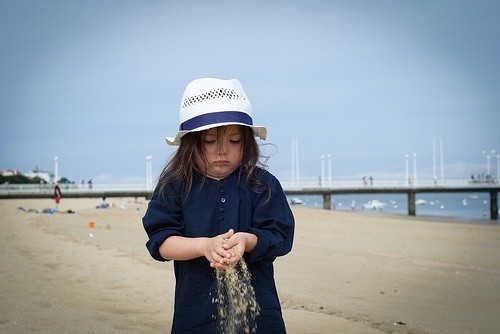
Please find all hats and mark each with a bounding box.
[165,77,267,146]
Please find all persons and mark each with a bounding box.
[142,78,295,334]
[361,175,375,187]
[481,206,500,224]
[55,185,62,212]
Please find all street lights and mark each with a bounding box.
[412,154,418,185]
[320,154,325,185]
[481,148,496,177]
[145,155,152,186]
[53,155,58,182]
[328,154,332,183]
[404,153,409,183]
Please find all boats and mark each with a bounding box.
[462,198,467,206]
[470,195,480,199]
[415,199,426,206]
[291,197,302,204]
[365,200,386,209]
